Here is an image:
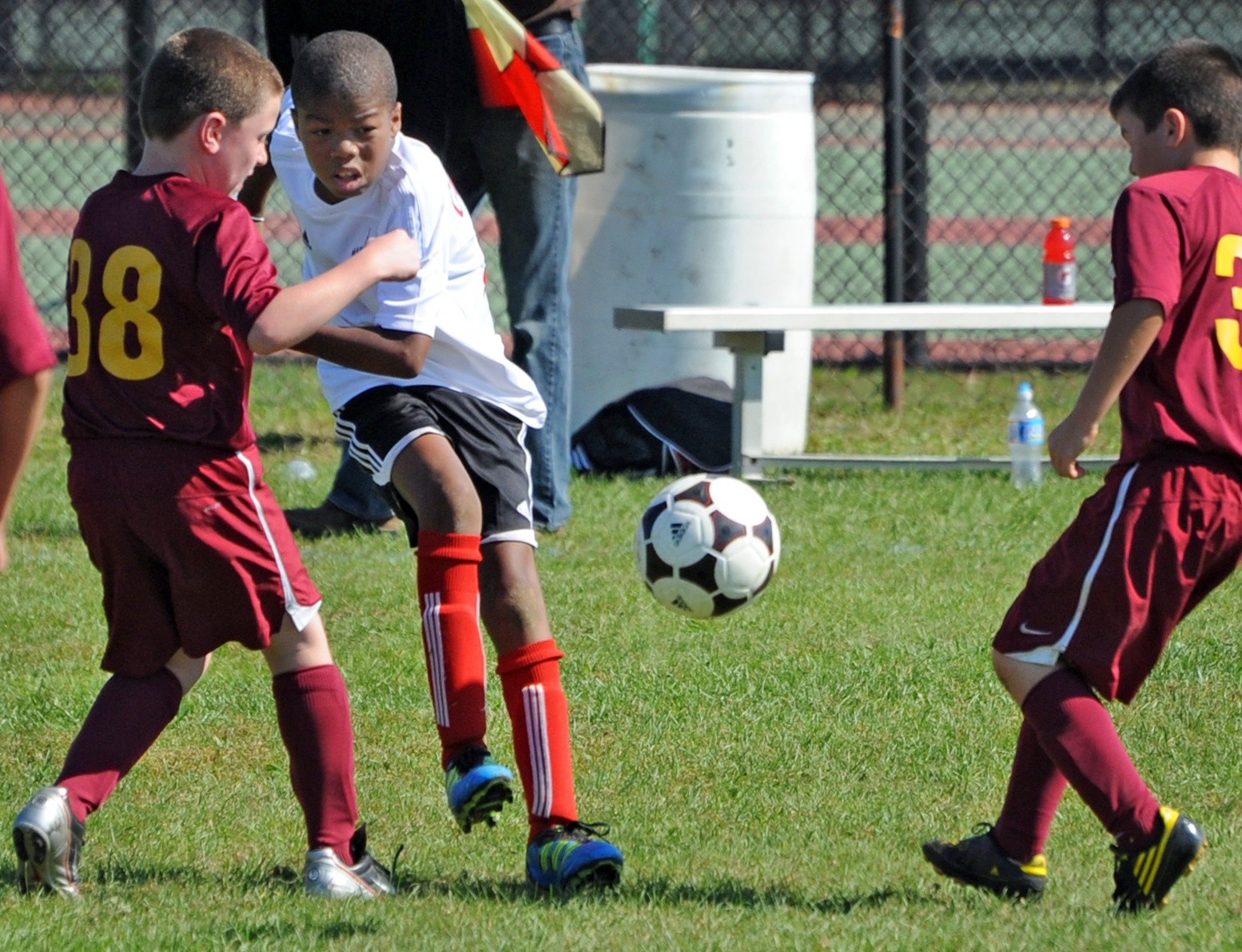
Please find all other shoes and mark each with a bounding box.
[282,499,393,538]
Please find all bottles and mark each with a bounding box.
[1006,382,1046,489]
[1043,216,1077,304]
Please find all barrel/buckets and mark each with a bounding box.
[567,52,818,454]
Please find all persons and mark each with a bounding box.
[267,30,627,900]
[8,24,410,902]
[914,35,1242,913]
[1,164,57,574]
[231,0,592,539]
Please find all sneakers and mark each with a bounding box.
[525,822,624,889]
[1107,807,1208,916]
[12,787,88,896]
[302,822,396,899]
[444,764,515,835]
[920,821,1048,900]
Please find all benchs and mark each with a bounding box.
[615,302,1118,476]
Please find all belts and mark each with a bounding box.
[527,14,565,37]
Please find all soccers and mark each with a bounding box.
[634,473,781,618]
